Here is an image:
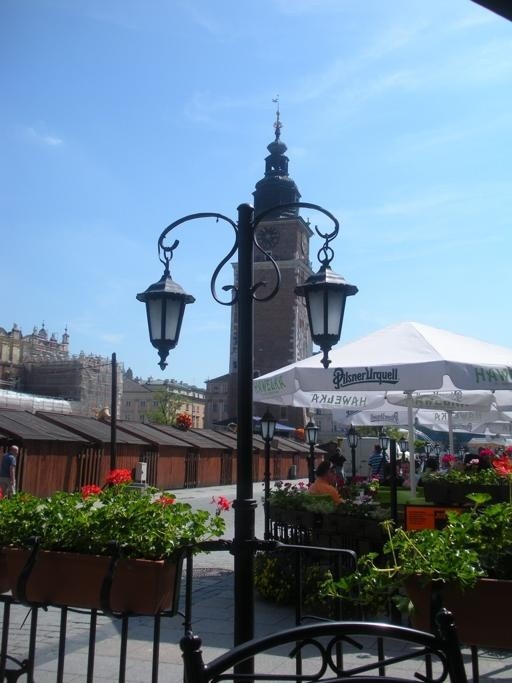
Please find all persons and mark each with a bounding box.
[0,445,20,496]
[305,460,347,507]
[367,443,411,488]
[414,445,511,486]
[329,447,347,489]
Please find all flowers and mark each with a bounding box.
[0,465,230,567]
[470,443,511,478]
[441,451,454,464]
[273,477,379,506]
[176,412,194,432]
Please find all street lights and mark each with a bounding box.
[346,423,465,484]
[133,200,360,681]
[302,415,320,490]
[258,404,276,541]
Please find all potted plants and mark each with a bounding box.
[421,467,500,505]
